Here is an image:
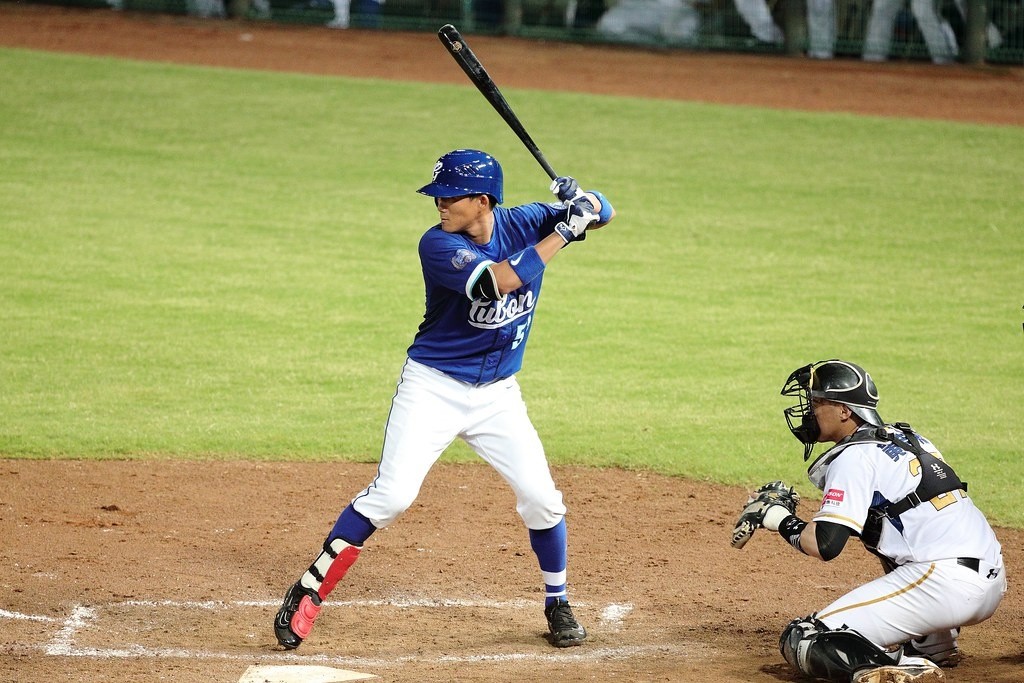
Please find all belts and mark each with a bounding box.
[957,556,998,580]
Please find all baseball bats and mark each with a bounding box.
[436,22,561,183]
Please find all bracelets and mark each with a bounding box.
[587,190,612,223]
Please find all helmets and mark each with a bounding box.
[811,359,884,427]
[416,148,504,205]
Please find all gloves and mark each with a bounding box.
[554,194,601,248]
[550,176,583,204]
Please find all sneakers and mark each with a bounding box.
[543,597,585,646]
[274,578,321,649]
[910,627,960,665]
[850,664,947,683]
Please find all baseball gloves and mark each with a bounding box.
[730,482,799,550]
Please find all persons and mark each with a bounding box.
[274,150,617,649]
[326,0,1000,65]
[731,360,1007,683]
